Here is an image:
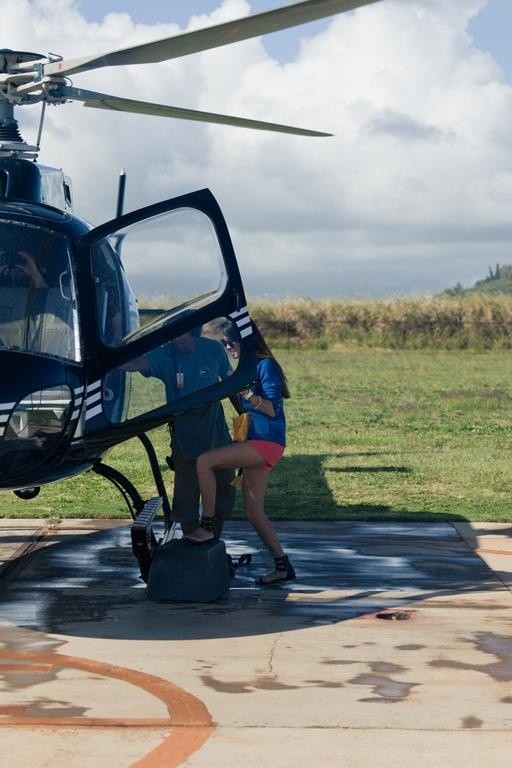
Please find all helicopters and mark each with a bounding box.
[0,1,387,586]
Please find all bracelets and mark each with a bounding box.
[254,396,261,410]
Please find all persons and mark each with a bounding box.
[192,317,297,587]
[105,311,235,540]
[0,237,56,287]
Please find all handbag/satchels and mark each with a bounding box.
[232,412,249,442]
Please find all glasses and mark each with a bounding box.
[221,338,238,347]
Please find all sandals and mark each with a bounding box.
[182,517,218,543]
[255,554,296,584]
